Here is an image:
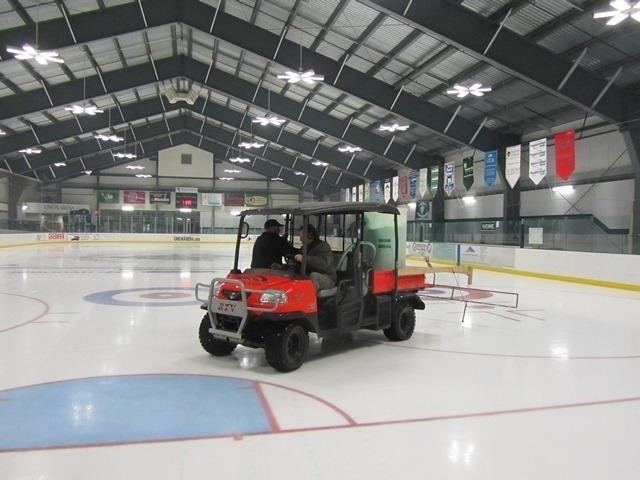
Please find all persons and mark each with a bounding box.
[294,223,338,294]
[251,218,300,270]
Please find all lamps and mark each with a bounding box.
[276,2,327,88]
[270,169,305,181]
[591,0,638,30]
[5,0,152,179]
[308,158,330,169]
[215,169,244,182]
[444,79,493,100]
[376,120,412,134]
[250,66,287,128]
[228,155,253,164]
[337,143,363,156]
[236,140,263,149]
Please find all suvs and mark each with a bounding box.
[191,200,429,371]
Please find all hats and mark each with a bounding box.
[264,219,284,228]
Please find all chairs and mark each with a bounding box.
[314,251,354,299]
